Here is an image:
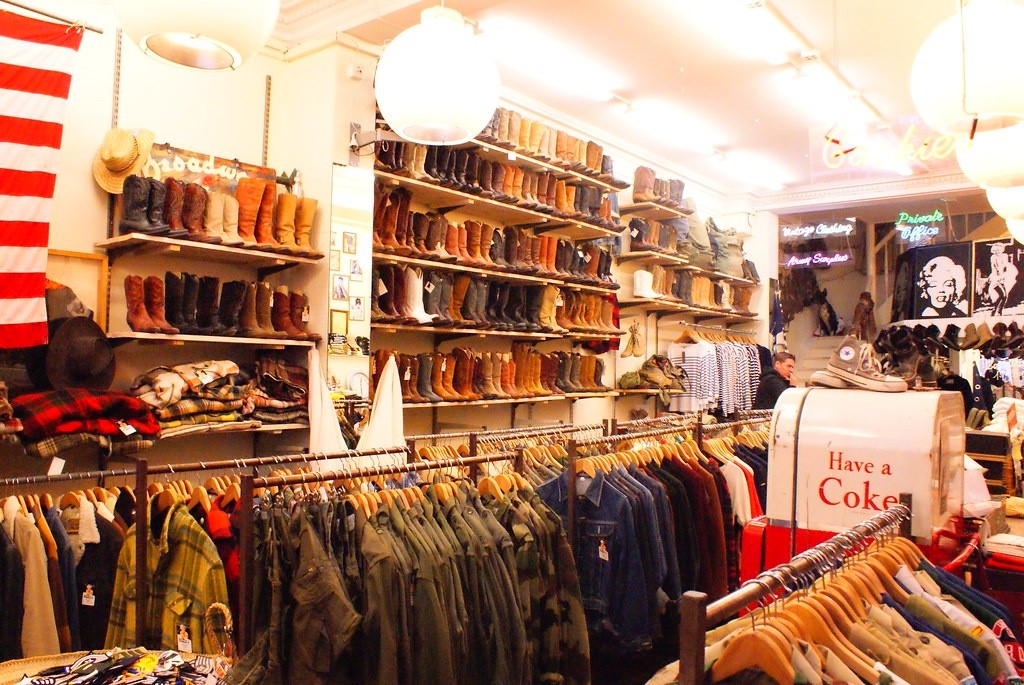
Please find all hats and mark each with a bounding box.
[92,127,150,194]
[45,316,116,390]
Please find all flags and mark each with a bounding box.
[0,12,85,348]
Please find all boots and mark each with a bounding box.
[873,322,1024,386]
[374,97,760,406]
[117,169,325,341]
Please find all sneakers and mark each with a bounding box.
[811,334,908,392]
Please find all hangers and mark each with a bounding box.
[0,408,773,534]
[956,543,981,586]
[673,322,761,347]
[703,504,936,685]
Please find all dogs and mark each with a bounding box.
[816,288,843,337]
[847,291,874,339]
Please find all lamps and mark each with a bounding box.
[374,0,499,145]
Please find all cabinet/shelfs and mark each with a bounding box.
[98,233,318,435]
[373,117,620,407]
[615,201,762,396]
[964,431,1024,501]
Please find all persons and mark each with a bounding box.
[752,351,797,409]
